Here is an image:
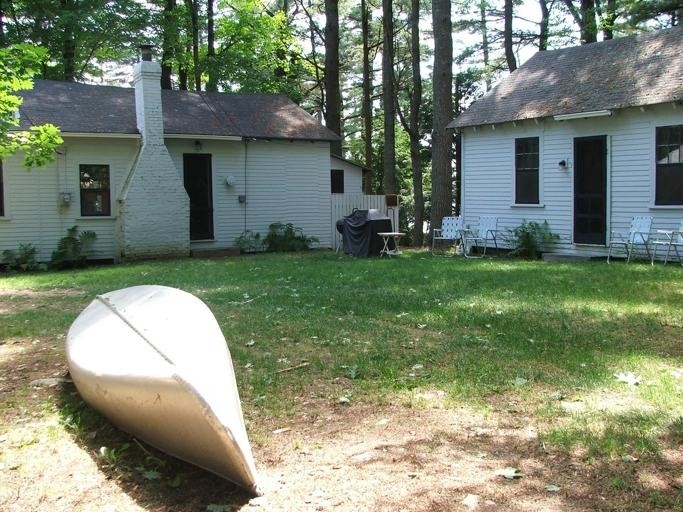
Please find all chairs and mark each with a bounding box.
[430,215,500,259]
[605,215,682,266]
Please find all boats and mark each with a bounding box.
[62,284,267,499]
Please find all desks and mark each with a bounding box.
[377,232,406,259]
